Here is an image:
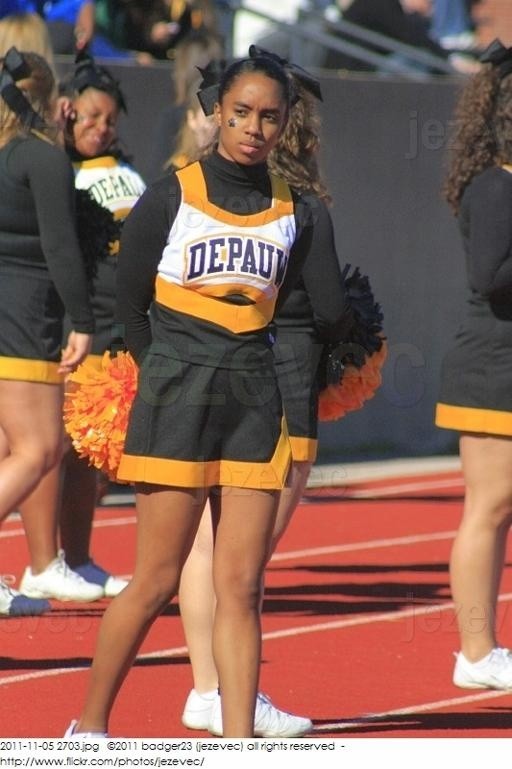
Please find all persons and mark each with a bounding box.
[0,0,512,738]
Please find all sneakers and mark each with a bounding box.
[2,575,53,618]
[60,718,110,737]
[209,692,313,737]
[71,561,130,599]
[451,643,512,693]
[180,687,220,733]
[17,556,104,604]
